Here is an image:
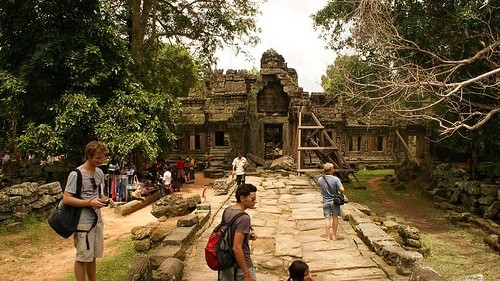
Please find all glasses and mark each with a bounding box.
[89,177,97,189]
[252,197,255,202]
[237,153,239,154]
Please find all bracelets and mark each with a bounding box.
[87,199,91,208]
[244,271,249,278]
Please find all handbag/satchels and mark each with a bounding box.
[334,195,344,205]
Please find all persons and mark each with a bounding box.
[116,154,195,196]
[287,260,312,281]
[217,184,258,281]
[62,141,114,281]
[231,149,247,186]
[0,149,65,166]
[202,153,211,169]
[317,163,344,241]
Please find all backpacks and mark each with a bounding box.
[48,168,98,250]
[205,207,249,281]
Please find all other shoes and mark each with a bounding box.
[322,238,330,242]
